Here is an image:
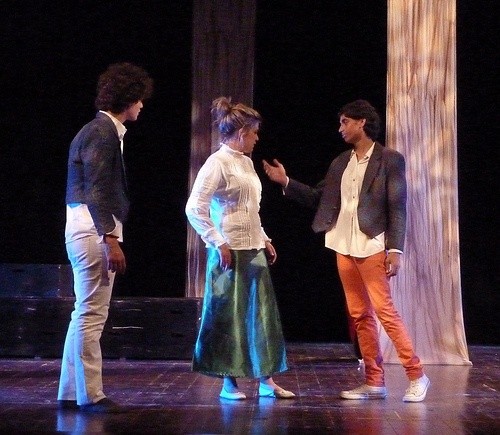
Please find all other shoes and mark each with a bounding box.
[259,387,295,398]
[220,387,246,399]
[80,398,122,413]
[60,400,79,409]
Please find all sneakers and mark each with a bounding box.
[339,384,386,400]
[403,374,430,401]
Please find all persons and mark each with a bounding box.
[185,97,295,400]
[57,62,154,413]
[262,100,431,402]
